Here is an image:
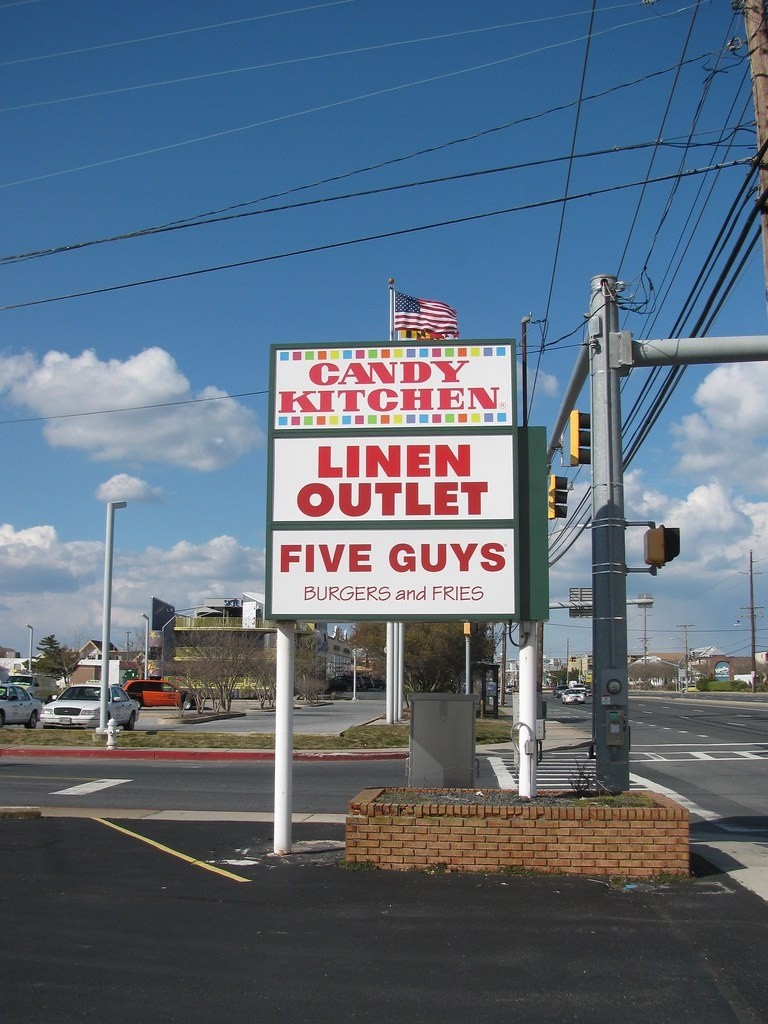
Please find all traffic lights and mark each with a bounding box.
[570,413,591,465]
[646,528,683,563]
[548,475,568,518]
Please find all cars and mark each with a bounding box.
[552,680,593,704]
[39,684,140,730]
[0,685,42,729]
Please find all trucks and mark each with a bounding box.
[0,675,59,705]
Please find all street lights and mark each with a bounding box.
[669,637,689,693]
[141,612,150,680]
[91,497,127,741]
[733,623,756,693]
[24,623,34,672]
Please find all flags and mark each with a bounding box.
[394,291,457,334]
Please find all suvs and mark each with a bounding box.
[123,680,202,710]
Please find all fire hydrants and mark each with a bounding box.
[102,717,124,750]
